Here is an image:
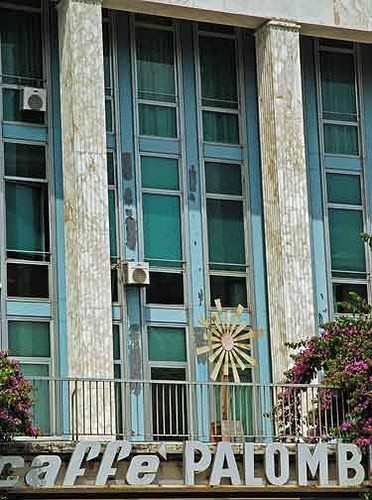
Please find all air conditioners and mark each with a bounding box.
[18,87,47,112]
[121,261,151,287]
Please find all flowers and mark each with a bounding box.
[0,350,43,442]
[260,289,370,456]
